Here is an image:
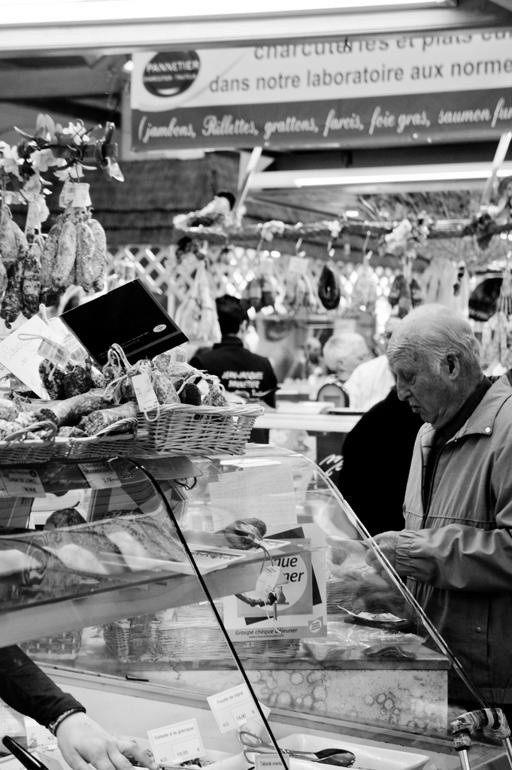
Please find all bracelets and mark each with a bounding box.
[48,708,81,737]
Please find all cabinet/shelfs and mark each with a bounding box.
[3,443,509,770]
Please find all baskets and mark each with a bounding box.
[119,359,267,463]
[0,420,60,468]
[53,417,140,464]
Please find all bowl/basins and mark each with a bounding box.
[301,638,350,659]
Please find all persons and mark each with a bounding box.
[178,295,277,442]
[362,302,512,718]
[334,385,423,588]
[286,337,329,381]
[342,312,404,410]
[306,328,370,402]
[0,643,160,770]
